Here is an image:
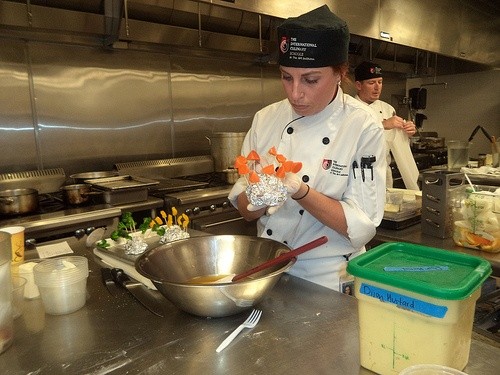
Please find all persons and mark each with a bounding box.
[354,61,417,188]
[227,4,387,294]
[344,285,352,295]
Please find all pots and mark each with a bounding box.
[206,131,248,172]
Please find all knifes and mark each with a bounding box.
[112,268,165,317]
[100,267,119,300]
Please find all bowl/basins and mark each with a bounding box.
[69,172,120,182]
[449,183,500,254]
[60,182,104,205]
[135,234,299,318]
[12,255,90,317]
[0,188,39,216]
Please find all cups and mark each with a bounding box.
[399,365,468,375]
[445,139,471,169]
[9,276,27,318]
[0,227,26,263]
[491,143,500,167]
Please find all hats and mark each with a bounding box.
[355,61,383,82]
[277,4,350,68]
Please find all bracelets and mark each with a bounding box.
[291,184,309,200]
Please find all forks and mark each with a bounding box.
[216,308,262,353]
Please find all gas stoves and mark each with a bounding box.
[169,168,255,223]
[0,184,104,245]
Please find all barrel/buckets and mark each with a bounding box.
[0,257,16,353]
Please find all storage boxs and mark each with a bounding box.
[346,242,492,375]
